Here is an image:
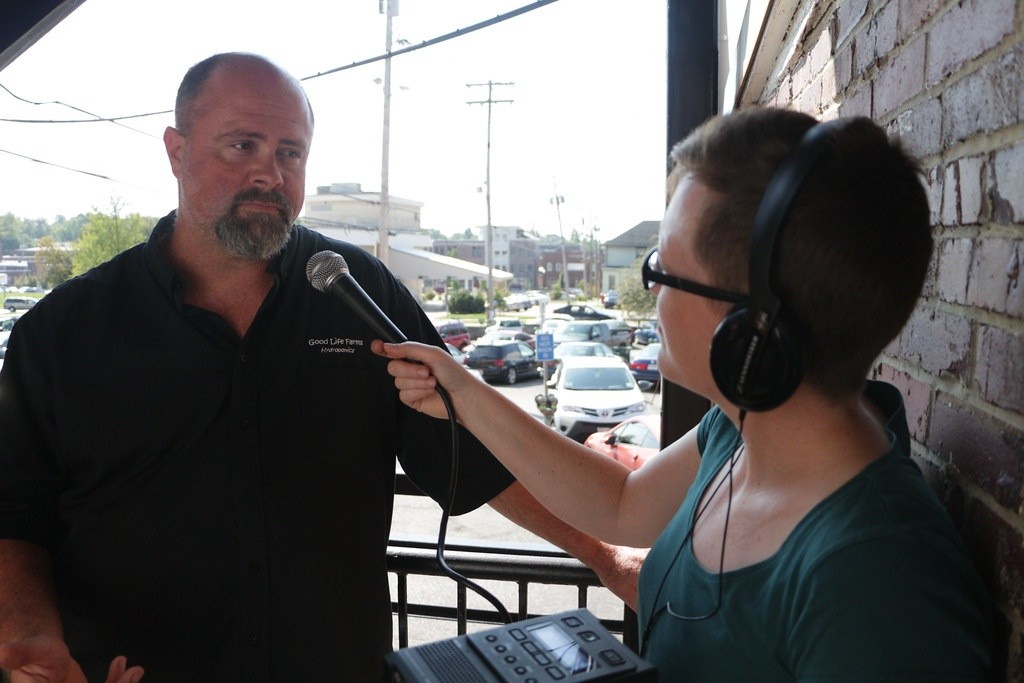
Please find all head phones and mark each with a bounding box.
[708,116,879,414]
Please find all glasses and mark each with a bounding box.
[641,247,749,308]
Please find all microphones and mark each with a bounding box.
[305,251,425,367]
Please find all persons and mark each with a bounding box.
[371,108,1012,683]
[0,51,650,683]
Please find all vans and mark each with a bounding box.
[4,297,38,313]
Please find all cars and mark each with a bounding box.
[485,305,660,349]
[537,342,622,380]
[435,320,471,350]
[629,343,661,384]
[546,356,646,445]
[464,340,544,385]
[583,415,661,472]
